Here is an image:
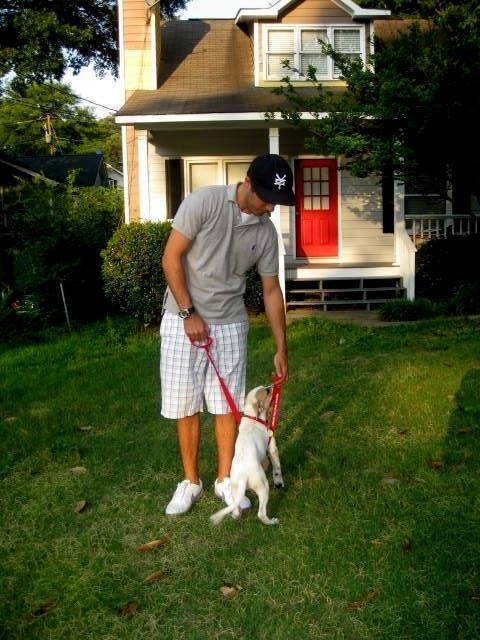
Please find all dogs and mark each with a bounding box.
[209,381,287,528]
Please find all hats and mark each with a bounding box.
[248,155,296,207]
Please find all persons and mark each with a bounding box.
[159,155,299,516]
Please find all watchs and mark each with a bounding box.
[179,307,195,319]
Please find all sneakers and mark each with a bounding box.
[165,477,203,516]
[215,477,251,509]
